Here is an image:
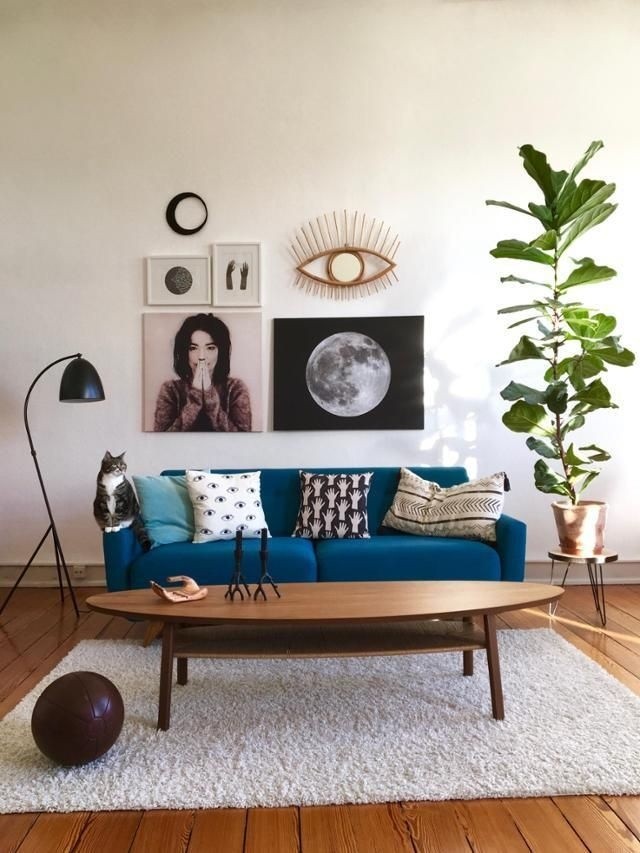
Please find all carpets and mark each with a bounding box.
[0,628,640,815]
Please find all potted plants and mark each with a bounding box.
[486,141,635,555]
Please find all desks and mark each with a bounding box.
[546,549,617,626]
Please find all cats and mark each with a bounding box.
[94,451,151,549]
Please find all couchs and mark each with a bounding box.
[102,467,526,624]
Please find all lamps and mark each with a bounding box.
[1,353,104,620]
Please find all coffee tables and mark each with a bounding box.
[83,583,566,730]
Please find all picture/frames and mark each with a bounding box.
[211,242,262,308]
[146,253,211,307]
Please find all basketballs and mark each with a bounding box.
[30,670,126,768]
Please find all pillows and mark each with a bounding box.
[184,470,272,543]
[127,469,210,548]
[291,470,374,539]
[381,467,510,543]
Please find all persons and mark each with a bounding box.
[154,313,252,434]
